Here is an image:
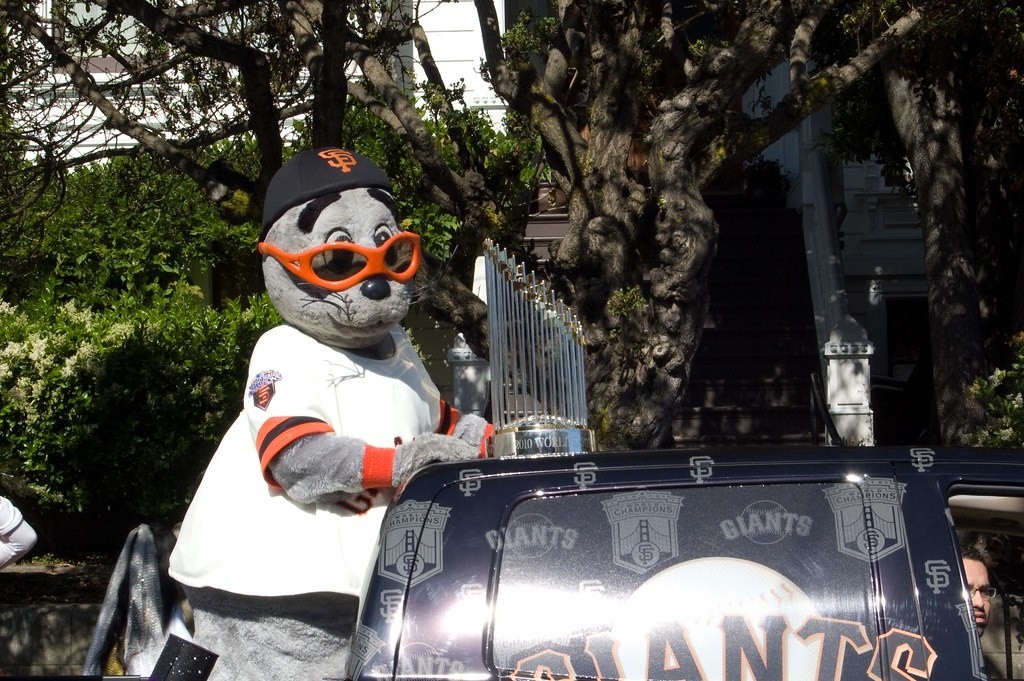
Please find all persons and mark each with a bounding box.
[955,538,1004,681]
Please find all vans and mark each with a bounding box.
[344,445,1023,681]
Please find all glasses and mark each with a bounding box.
[967,586,997,598]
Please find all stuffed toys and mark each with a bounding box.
[168,149,503,681]
